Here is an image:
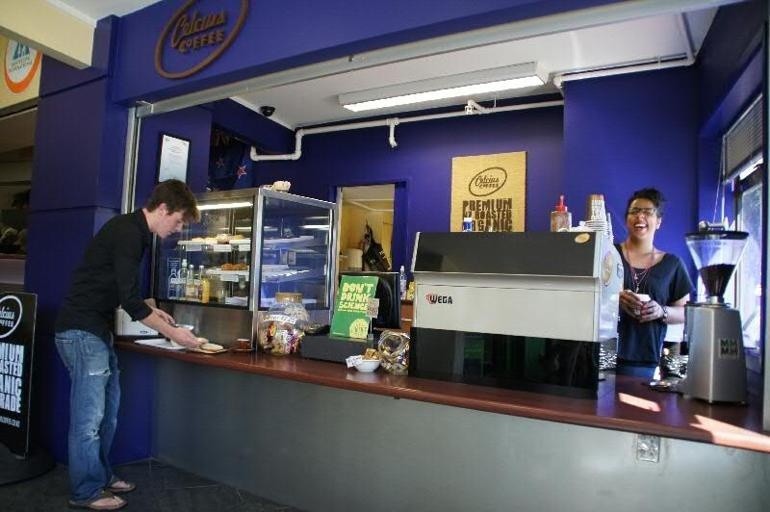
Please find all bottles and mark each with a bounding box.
[463,216,473,232]
[550,194,572,233]
[197,265,210,304]
[186,264,198,301]
[399,265,407,301]
[377,329,411,376]
[175,258,187,301]
[256,291,312,357]
[167,267,177,300]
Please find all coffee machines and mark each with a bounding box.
[669,229,750,406]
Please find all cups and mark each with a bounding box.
[585,192,615,242]
[632,293,651,321]
[237,338,251,349]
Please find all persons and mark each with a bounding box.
[614,187,694,381]
[53,179,201,510]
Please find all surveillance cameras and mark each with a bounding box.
[261,106,275,116]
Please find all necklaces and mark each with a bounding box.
[624,241,654,293]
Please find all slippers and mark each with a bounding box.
[69,476,136,510]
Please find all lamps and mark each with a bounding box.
[338,62,549,113]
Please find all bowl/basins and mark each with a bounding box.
[353,354,381,373]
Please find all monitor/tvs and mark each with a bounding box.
[338,271,401,329]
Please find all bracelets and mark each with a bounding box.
[658,305,669,326]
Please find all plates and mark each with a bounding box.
[134,337,187,350]
[232,347,256,353]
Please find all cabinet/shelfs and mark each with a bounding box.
[148,188,339,349]
